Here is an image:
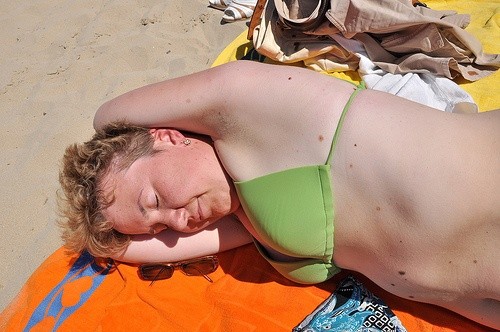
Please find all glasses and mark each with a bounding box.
[139,255,219,286]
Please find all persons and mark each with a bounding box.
[55,60,500,331]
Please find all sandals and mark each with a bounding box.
[209,0,258,23]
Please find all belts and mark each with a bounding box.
[283,0,329,32]
